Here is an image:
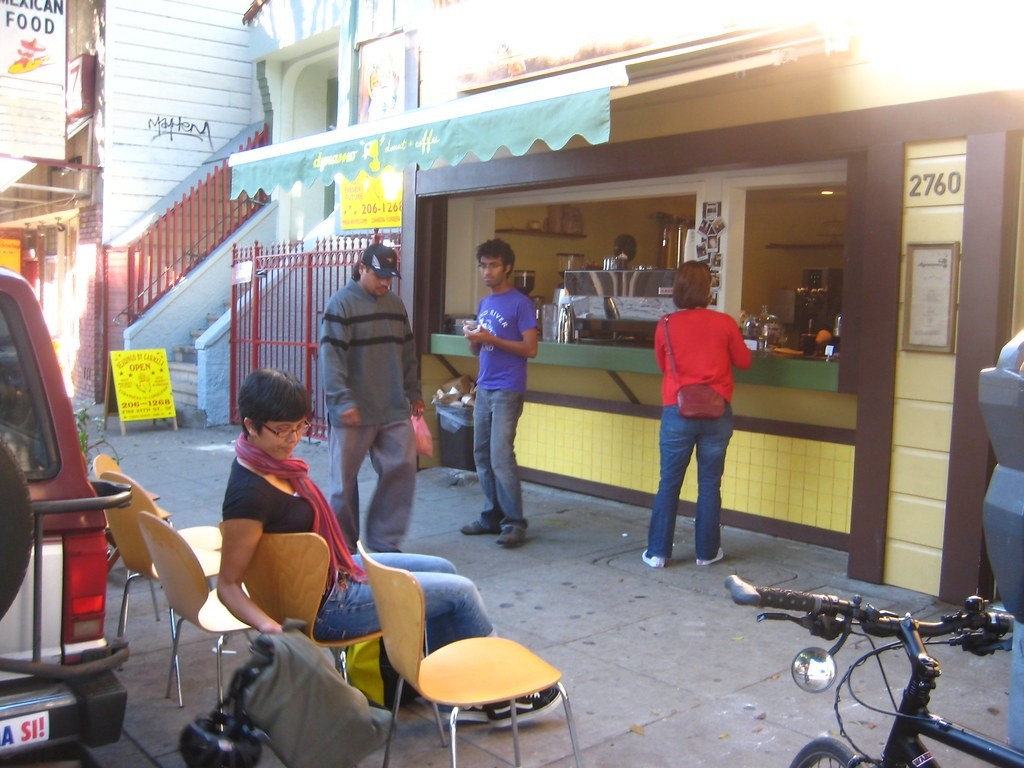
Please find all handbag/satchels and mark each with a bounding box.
[231,618,396,768]
[412,413,433,457]
[677,383,726,420]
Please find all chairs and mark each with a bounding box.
[355,538,583,768]
[94,452,230,707]
[215,520,448,768]
[132,510,257,717]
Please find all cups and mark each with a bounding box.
[542,288,563,342]
[801,333,817,356]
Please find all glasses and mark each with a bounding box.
[476,262,510,271]
[260,419,312,438]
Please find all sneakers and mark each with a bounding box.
[439,703,491,723]
[485,685,564,728]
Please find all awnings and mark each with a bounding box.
[227,31,858,200]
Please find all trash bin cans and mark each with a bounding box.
[436,404,476,471]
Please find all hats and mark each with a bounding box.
[361,242,401,279]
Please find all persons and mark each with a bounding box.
[319,244,427,552]
[461,241,538,544]
[643,260,751,569]
[217,371,566,727]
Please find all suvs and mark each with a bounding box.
[0,267,133,767]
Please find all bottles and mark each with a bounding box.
[557,296,575,344]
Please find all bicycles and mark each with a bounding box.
[179,619,393,768]
[723,574,1024,768]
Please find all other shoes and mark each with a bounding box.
[643,549,666,568]
[460,521,501,534]
[696,546,724,566]
[496,530,526,545]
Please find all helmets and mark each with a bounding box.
[181,718,262,768]
